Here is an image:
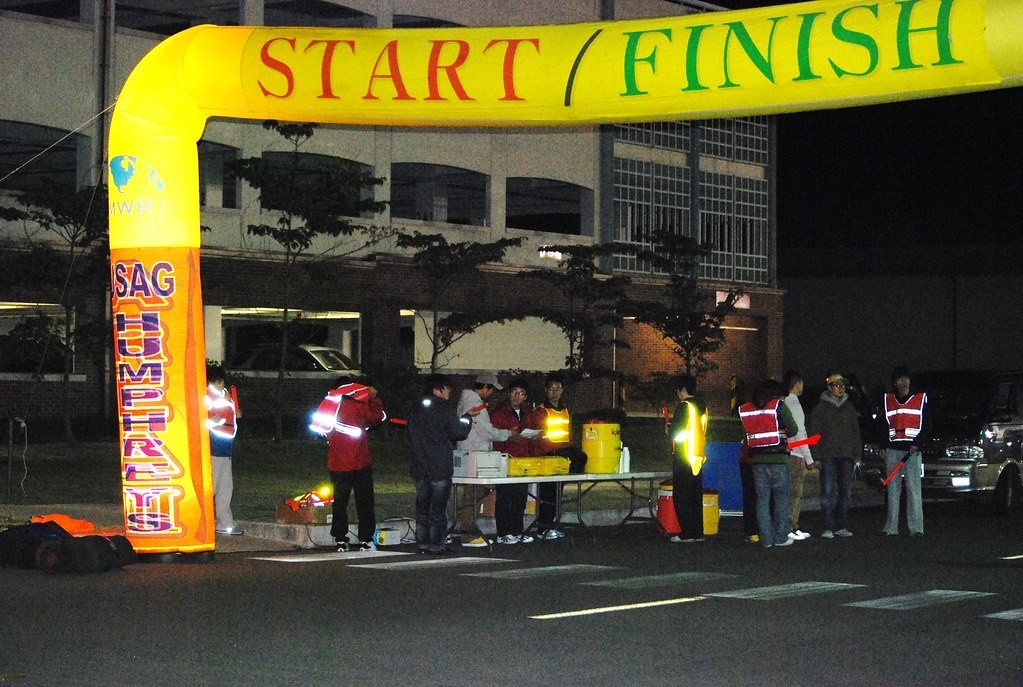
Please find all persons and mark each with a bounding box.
[457,371,520,548]
[876,365,930,538]
[489,379,534,545]
[737,371,798,548]
[206,367,244,534]
[404,380,480,552]
[808,373,864,539]
[532,375,574,540]
[784,373,814,541]
[309,376,388,553]
[665,374,708,543]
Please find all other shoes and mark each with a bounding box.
[428,542,447,551]
[418,540,429,550]
[774,537,795,546]
[764,544,773,547]
[670,536,693,543]
[877,531,899,537]
[694,537,706,542]
[912,532,924,541]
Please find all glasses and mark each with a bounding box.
[546,387,564,394]
[829,384,845,389]
[511,389,526,397]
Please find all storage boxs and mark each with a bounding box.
[658,479,720,534]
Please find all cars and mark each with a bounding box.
[220,340,363,388]
[855,367,1023,518]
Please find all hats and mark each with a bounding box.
[826,374,849,387]
[476,371,504,390]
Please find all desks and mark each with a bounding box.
[447,470,674,555]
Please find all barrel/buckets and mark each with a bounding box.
[702,488,720,534]
[656,479,684,534]
[582,423,621,475]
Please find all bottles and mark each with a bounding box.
[623,447,630,474]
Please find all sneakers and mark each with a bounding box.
[358,541,378,551]
[835,528,853,537]
[461,537,494,547]
[443,532,451,544]
[514,535,534,543]
[215,526,244,535]
[497,534,519,544]
[821,529,834,539]
[336,541,350,551]
[537,529,566,539]
[787,529,811,540]
